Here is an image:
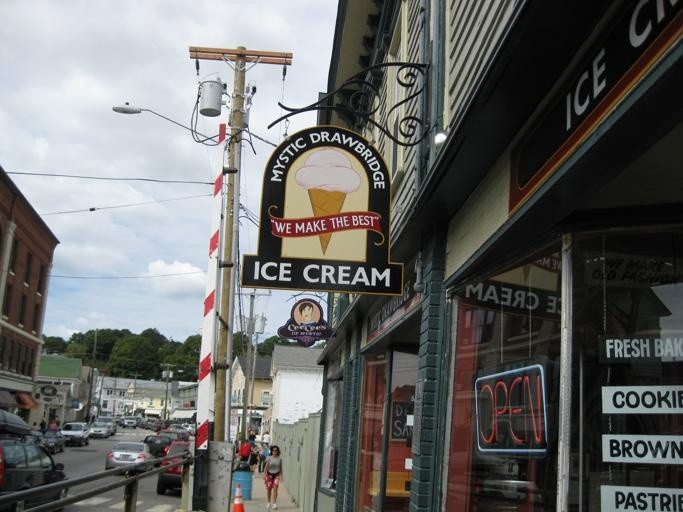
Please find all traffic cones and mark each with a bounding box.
[232,483,247,512]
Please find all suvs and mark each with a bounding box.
[0,411,69,511]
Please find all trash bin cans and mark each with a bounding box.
[231,461,253,500]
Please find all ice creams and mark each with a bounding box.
[296,149,362,255]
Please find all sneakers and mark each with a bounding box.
[265,503,277,510]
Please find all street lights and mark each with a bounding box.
[110,103,249,445]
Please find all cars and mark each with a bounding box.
[41,414,196,497]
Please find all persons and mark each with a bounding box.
[263,445,283,510]
[31,418,59,435]
[156,419,162,435]
[237,427,270,474]
